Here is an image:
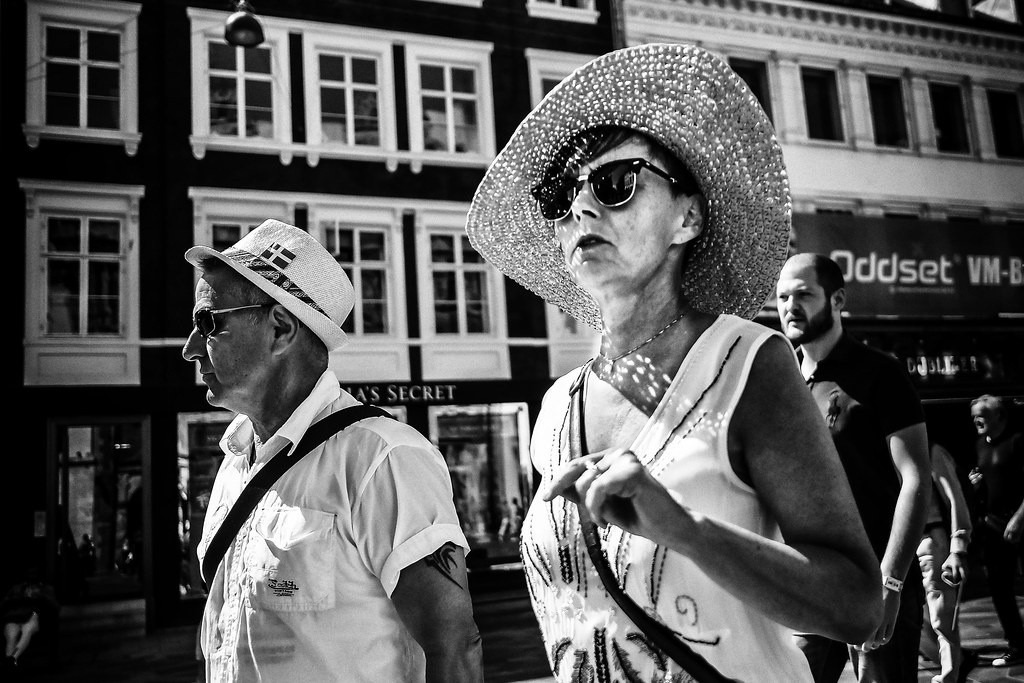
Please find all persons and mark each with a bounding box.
[182,219,483,683]
[465,43,882,683]
[0,567,59,666]
[776,252,1024,683]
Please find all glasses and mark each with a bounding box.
[530,158,682,221]
[193,304,270,338]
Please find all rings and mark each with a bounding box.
[585,464,602,478]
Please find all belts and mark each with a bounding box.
[923,521,947,534]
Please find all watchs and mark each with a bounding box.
[882,575,903,592]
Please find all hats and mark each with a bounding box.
[184,218,355,353]
[466,43,793,333]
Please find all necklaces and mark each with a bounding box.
[599,306,692,374]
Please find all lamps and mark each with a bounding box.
[225,0,265,49]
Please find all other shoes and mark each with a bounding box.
[957,648,978,683]
[992,648,1024,666]
